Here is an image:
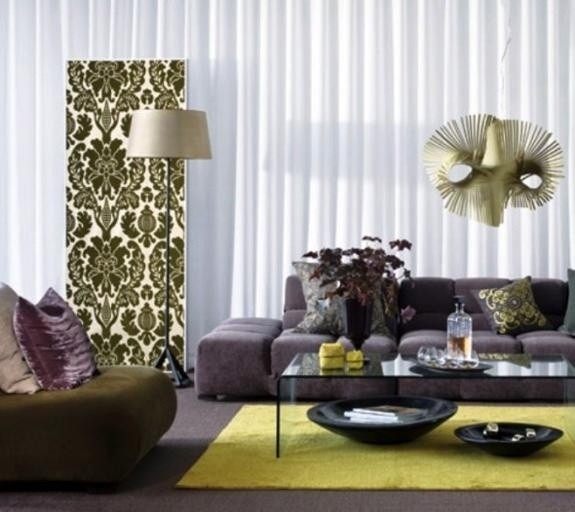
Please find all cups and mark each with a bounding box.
[416,344,478,369]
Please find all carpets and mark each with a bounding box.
[174,402,575,492]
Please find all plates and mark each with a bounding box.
[418,362,493,374]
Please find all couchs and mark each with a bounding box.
[270,274,574,402]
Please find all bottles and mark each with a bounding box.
[445,301,473,358]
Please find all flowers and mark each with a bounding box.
[302,236,417,323]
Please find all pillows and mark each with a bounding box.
[0,282,46,396]
[291,261,397,333]
[12,287,97,392]
[557,268,575,334]
[469,275,555,335]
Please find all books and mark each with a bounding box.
[344,405,427,423]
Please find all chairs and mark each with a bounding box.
[0,359,177,492]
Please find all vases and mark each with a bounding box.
[344,297,374,350]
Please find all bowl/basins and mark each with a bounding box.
[306,393,458,447]
[454,420,565,452]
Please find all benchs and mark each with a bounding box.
[193,317,283,400]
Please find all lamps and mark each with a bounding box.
[126,109,213,388]
